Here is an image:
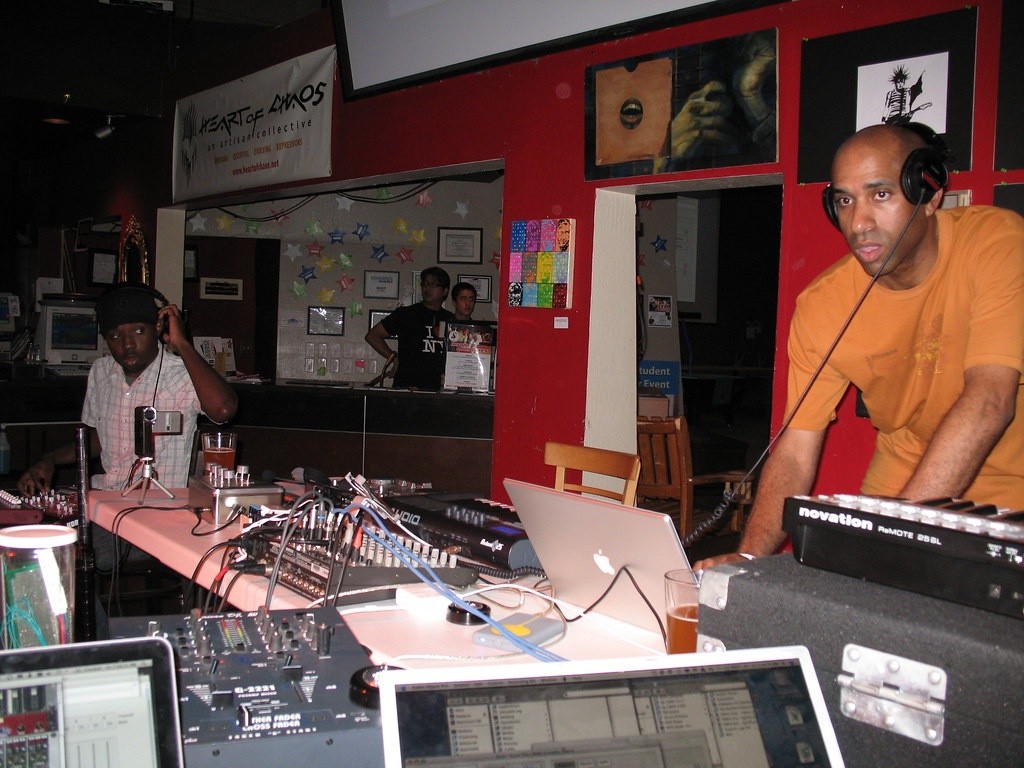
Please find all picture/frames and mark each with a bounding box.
[459,275,492,302]
[583,28,780,181]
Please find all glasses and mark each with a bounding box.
[420,281,443,288]
[455,295,476,303]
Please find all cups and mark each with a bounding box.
[202,432,236,476]
[663,569,703,653]
[214,352,228,379]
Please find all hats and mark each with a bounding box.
[93,282,158,334]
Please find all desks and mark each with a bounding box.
[89,490,329,612]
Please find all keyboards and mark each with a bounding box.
[54,368,90,376]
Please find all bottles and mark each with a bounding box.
[0,524,78,651]
[210,464,250,488]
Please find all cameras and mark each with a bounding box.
[134,406,181,457]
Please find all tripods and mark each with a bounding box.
[121,458,175,505]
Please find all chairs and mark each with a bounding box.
[545,443,640,507]
[638,418,754,542]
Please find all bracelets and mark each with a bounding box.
[737,551,756,561]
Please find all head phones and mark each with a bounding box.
[97,281,172,345]
[822,121,957,233]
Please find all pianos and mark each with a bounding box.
[377,491,547,575]
[782,494,1024,617]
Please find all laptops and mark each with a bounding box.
[0,636,185,768]
[502,476,700,635]
[375,644,846,768]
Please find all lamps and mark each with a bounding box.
[94,114,125,141]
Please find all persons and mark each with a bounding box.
[452,283,477,321]
[364,266,456,391]
[692,124,1024,584]
[18,282,239,641]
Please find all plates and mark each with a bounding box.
[42,293,99,301]
[36,300,100,305]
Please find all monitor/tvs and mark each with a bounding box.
[34,306,104,365]
